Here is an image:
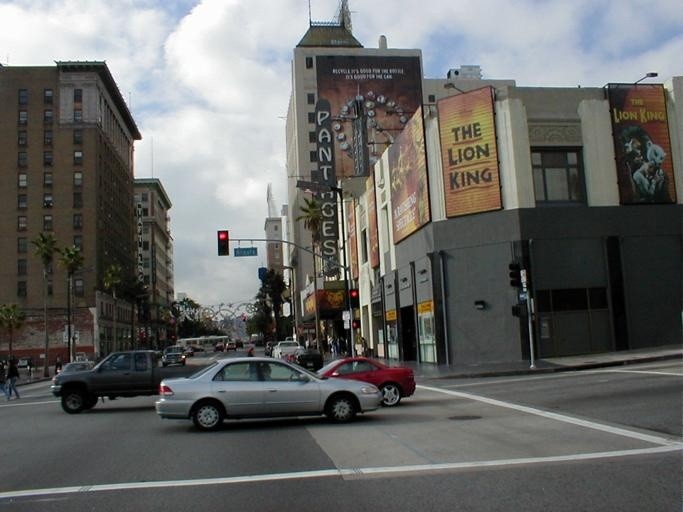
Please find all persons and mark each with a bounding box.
[248,347,254,356]
[327,335,347,358]
[267,345,275,358]
[361,337,367,356]
[0,359,20,401]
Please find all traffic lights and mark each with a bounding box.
[508,262,522,289]
[352,319,360,329]
[349,288,359,309]
[217,230,229,256]
[513,305,526,316]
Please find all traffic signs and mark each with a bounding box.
[234,247,257,257]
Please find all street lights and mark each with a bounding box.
[297,179,357,362]
[277,265,296,336]
